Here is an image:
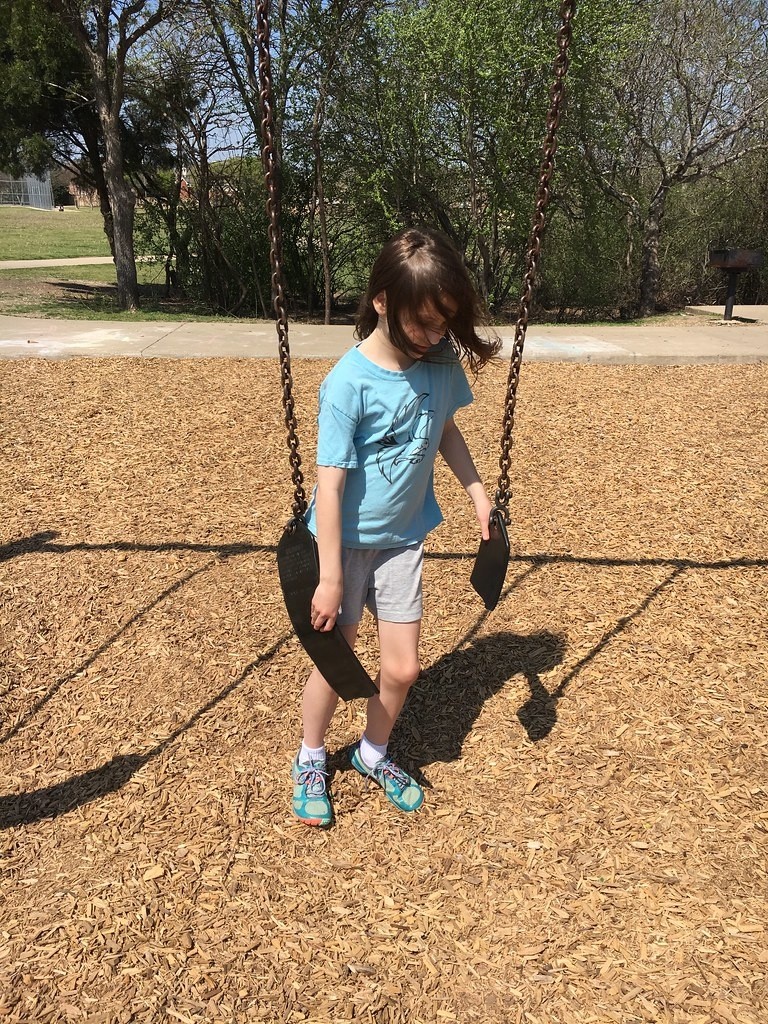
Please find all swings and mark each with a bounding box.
[254,1,580,702]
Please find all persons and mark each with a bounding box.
[59,204,64,211]
[291,227,504,828]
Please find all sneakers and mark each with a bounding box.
[350,746,424,812]
[292,748,331,826]
